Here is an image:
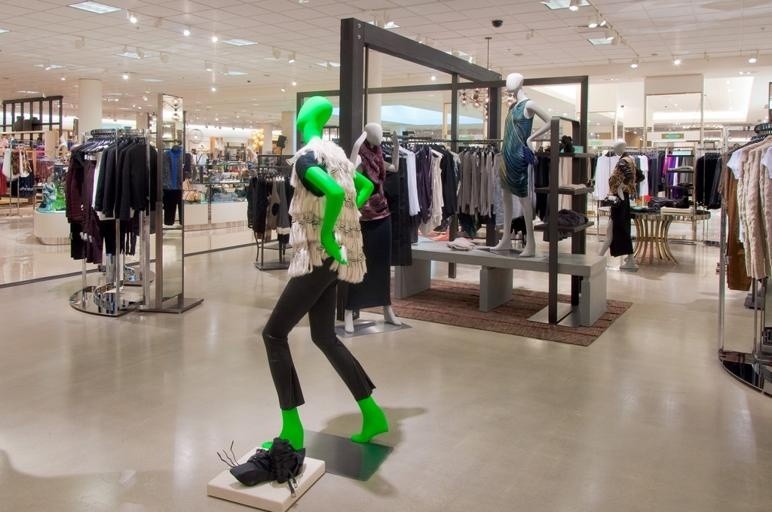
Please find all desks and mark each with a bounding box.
[598,206,711,265]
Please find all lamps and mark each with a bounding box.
[460,37,514,119]
[569,0,640,68]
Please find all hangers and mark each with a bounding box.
[255,165,292,182]
[729,123,772,155]
[73,129,146,154]
[382,134,495,158]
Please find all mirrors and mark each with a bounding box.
[140,93,204,315]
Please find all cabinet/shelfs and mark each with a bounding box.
[664,146,696,202]
[528,116,594,326]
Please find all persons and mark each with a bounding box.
[608,138,646,267]
[489,72,551,257]
[264,95,389,450]
[343,122,401,334]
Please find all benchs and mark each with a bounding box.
[396,240,607,327]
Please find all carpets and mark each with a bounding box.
[359,278,632,347]
